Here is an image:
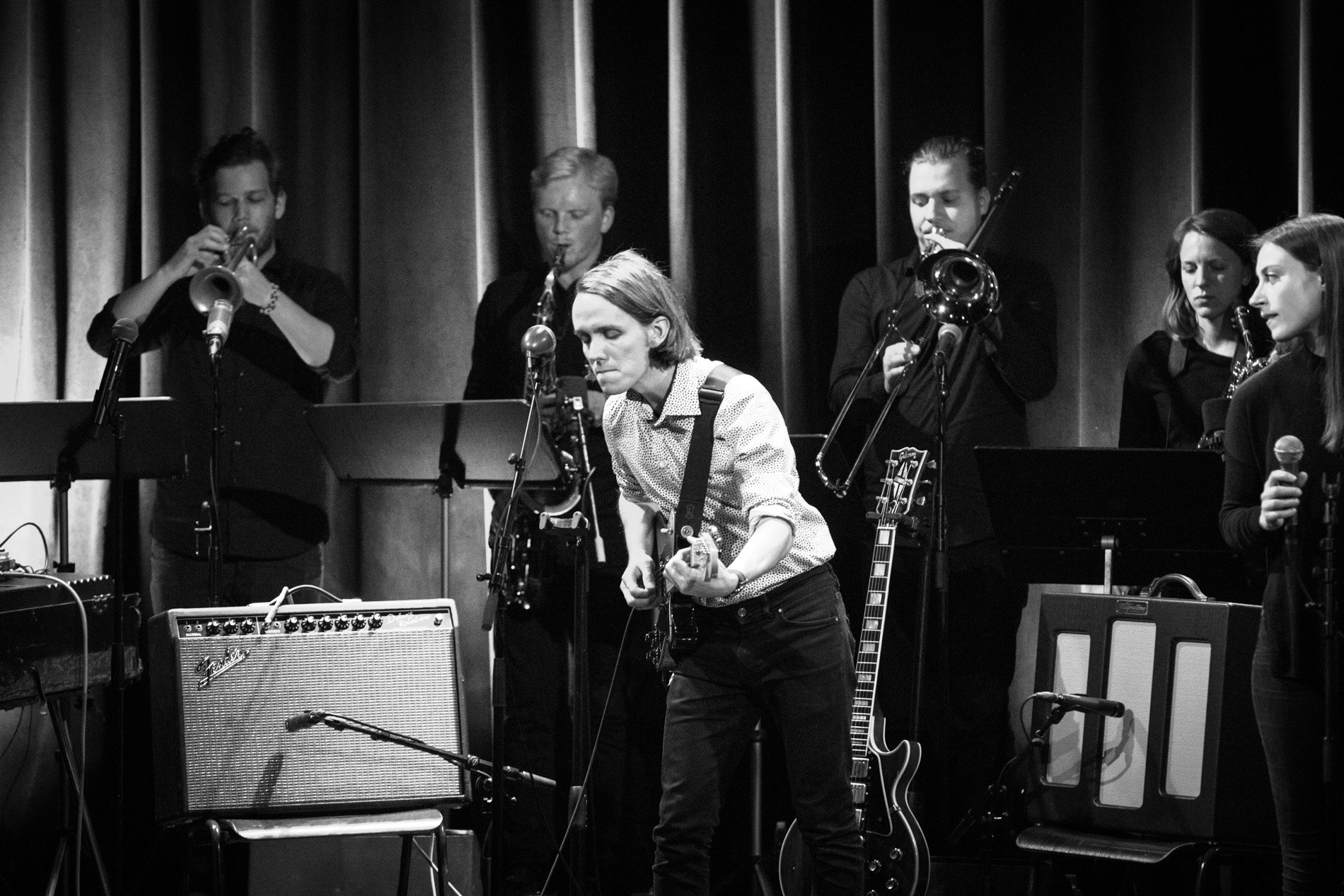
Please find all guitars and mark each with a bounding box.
[777,446,940,895]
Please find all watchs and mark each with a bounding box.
[723,570,748,599]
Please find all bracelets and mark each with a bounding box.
[258,282,280,315]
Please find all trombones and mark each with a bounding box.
[813,164,1022,498]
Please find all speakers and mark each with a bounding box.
[145,598,473,822]
[926,855,1036,896]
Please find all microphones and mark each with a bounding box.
[285,712,329,732]
[520,325,557,370]
[1035,693,1125,718]
[1273,436,1305,536]
[1233,305,1253,349]
[933,323,963,368]
[1200,397,1232,446]
[90,318,138,442]
[203,298,233,359]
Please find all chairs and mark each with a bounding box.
[1016,823,1231,896]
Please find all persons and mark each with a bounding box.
[831,136,1057,858]
[1118,207,1274,448]
[88,128,364,848]
[572,249,866,895]
[1220,216,1343,895]
[463,148,661,896]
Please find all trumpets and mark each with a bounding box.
[187,226,258,318]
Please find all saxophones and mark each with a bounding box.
[522,243,567,400]
[1194,306,1270,449]
